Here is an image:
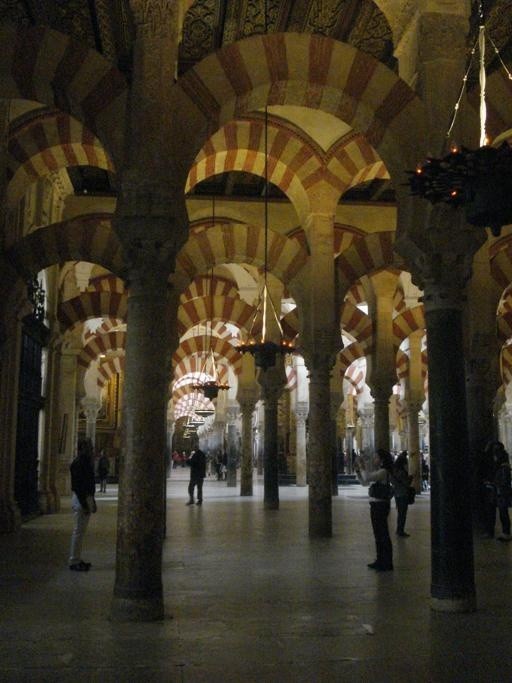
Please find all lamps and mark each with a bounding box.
[396,0,510,236]
[191,105,296,402]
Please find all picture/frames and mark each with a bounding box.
[78,354,119,430]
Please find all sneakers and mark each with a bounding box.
[69,559,91,572]
[397,531,410,536]
[187,499,202,504]
[368,561,394,570]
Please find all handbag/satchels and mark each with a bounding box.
[408,487,416,504]
[369,481,394,499]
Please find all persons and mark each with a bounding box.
[68,436,97,573]
[97,447,110,493]
[171,445,229,481]
[185,443,206,506]
[390,453,419,537]
[352,448,397,571]
[472,437,512,542]
[343,445,431,500]
